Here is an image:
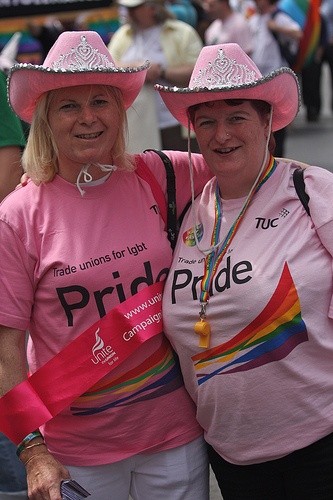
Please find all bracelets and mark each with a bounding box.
[16,432,46,457]
[159,64,165,80]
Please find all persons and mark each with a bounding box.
[0,0,333,500]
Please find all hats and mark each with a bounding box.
[116,0,144,7]
[154,43,300,132]
[7,30,150,124]
